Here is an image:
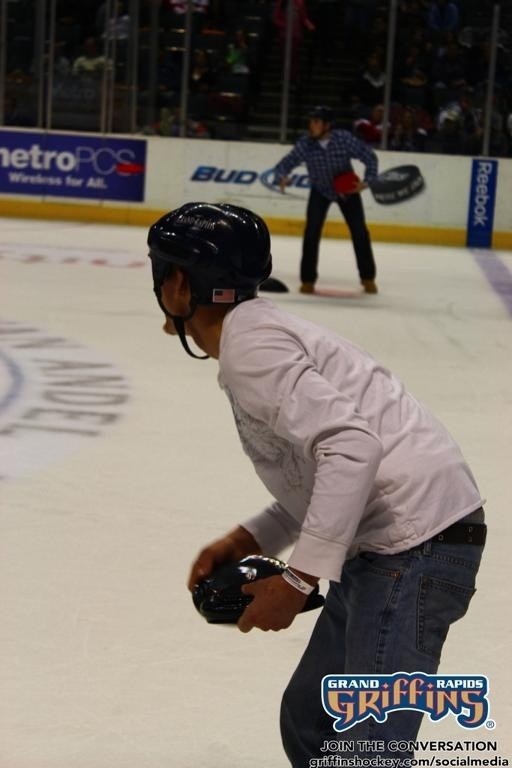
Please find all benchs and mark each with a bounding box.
[28,72,362,144]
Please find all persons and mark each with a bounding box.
[145,199,488,767]
[269,101,378,297]
[0,0,512,155]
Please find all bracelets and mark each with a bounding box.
[280,568,315,597]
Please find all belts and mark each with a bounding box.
[432,521,486,546]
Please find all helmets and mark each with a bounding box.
[147,202,271,322]
[308,107,333,122]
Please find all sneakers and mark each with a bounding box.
[301,282,312,293]
[362,279,377,293]
